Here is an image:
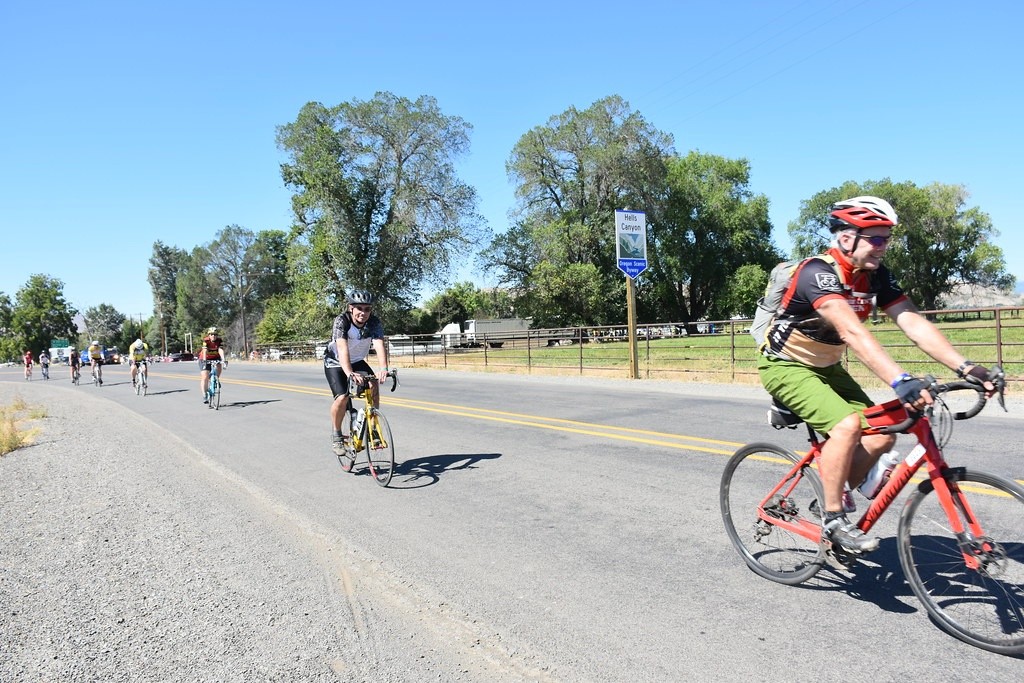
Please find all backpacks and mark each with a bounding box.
[751,254,848,351]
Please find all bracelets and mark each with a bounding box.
[380,368,387,371]
[957,361,971,374]
[890,373,908,388]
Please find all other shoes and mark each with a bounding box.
[142,384,147,388]
[100,380,103,384]
[72,380,74,383]
[132,381,135,387]
[91,371,94,376]
[216,382,221,388]
[203,396,209,403]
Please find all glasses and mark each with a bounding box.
[94,345,98,346]
[852,233,893,246]
[354,306,372,312]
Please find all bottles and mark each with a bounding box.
[357,409,365,422]
[860,450,899,499]
[841,480,856,513]
[352,408,358,431]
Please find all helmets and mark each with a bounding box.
[92,341,99,345]
[349,290,372,305]
[208,327,218,335]
[828,196,898,234]
[71,347,76,350]
[41,350,47,353]
[27,351,31,355]
[134,339,143,349]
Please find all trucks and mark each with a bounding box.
[441,319,534,349]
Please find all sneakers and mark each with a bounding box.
[368,430,379,441]
[821,517,880,553]
[331,433,346,456]
[808,499,821,518]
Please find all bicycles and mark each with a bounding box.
[331,368,403,486]
[67,363,83,386]
[201,358,229,411]
[40,363,49,381]
[718,368,1024,658]
[25,363,34,381]
[131,359,153,396]
[91,359,106,388]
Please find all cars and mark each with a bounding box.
[105,347,122,366]
[81,345,111,365]
[169,353,195,363]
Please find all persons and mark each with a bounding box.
[24,351,33,379]
[39,350,49,379]
[69,347,82,383]
[129,339,149,388]
[88,341,105,384]
[323,289,389,456]
[198,327,226,404]
[758,195,997,556]
